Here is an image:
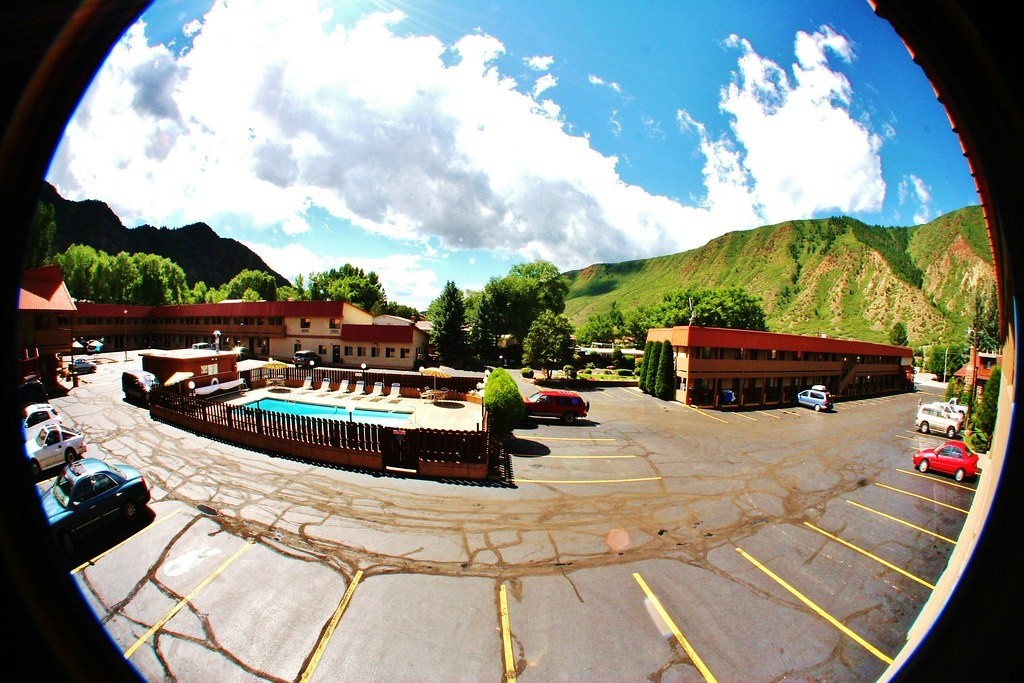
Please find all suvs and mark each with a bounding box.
[792,384,834,412]
[522,388,590,426]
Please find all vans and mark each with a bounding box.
[121,369,160,407]
[192,342,210,350]
[917,397,969,437]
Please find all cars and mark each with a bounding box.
[23,402,77,442]
[232,346,249,361]
[25,423,87,478]
[911,441,979,482]
[68,358,97,375]
[292,349,321,369]
[43,457,151,553]
